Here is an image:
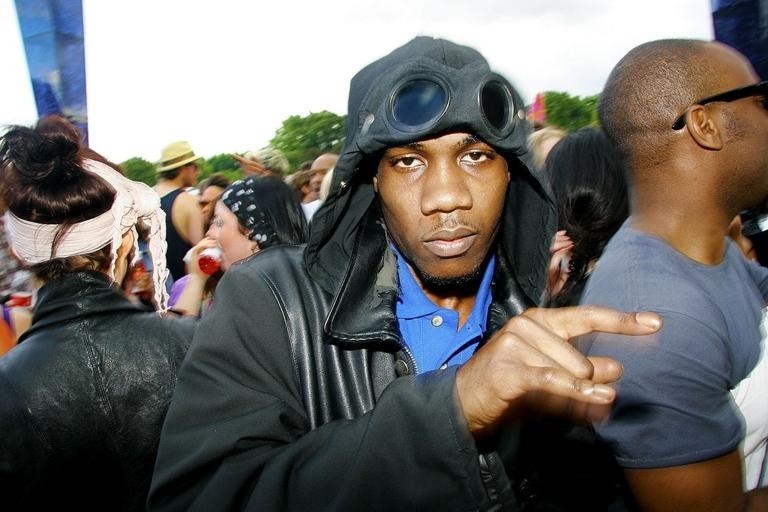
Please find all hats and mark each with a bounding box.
[156,141,201,172]
[306,32,559,320]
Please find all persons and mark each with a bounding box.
[0,36,768,510]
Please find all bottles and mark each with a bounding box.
[197,244,222,274]
